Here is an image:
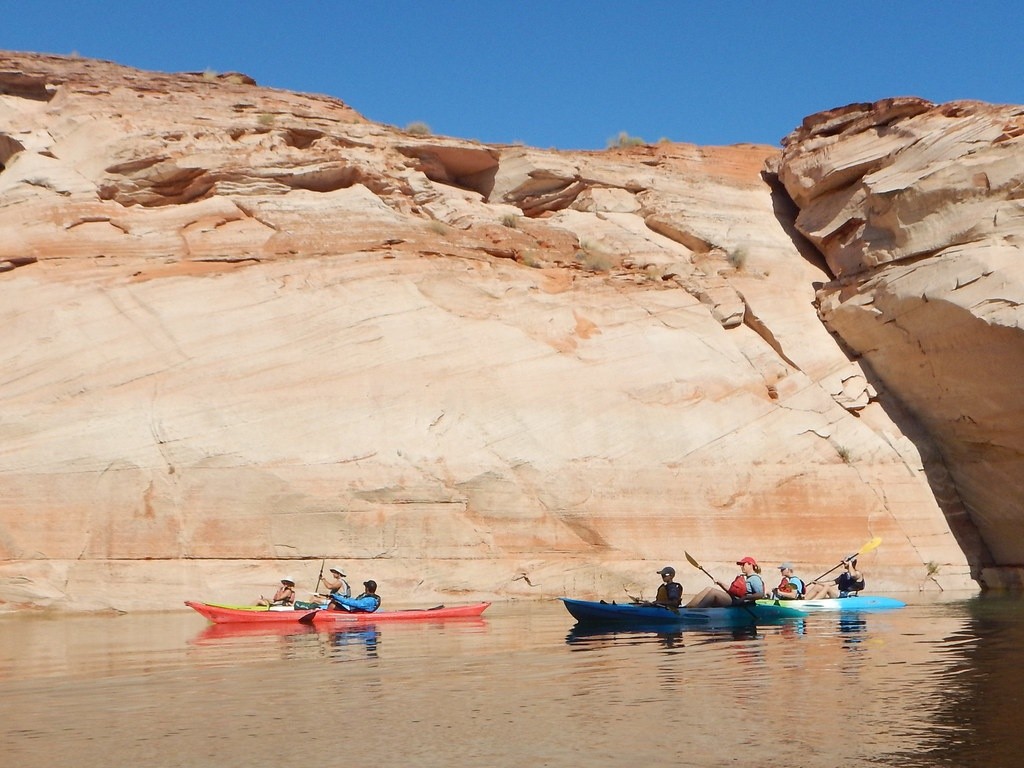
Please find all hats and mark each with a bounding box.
[841,555,857,561]
[776,562,793,569]
[281,575,296,586]
[330,566,347,577]
[737,557,757,566]
[657,567,676,575]
[364,580,377,592]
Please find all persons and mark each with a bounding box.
[686,557,765,608]
[309,568,351,604]
[258,577,295,606]
[328,581,380,611]
[600,567,683,605]
[765,563,802,600]
[805,559,863,600]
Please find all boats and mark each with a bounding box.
[309,600,492,628]
[751,594,910,621]
[554,591,809,630]
[182,596,317,626]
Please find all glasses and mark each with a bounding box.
[662,574,670,577]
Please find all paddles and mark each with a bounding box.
[683,550,737,599]
[313,556,327,592]
[766,583,798,597]
[806,536,883,586]
[626,593,680,616]
[312,587,335,599]
